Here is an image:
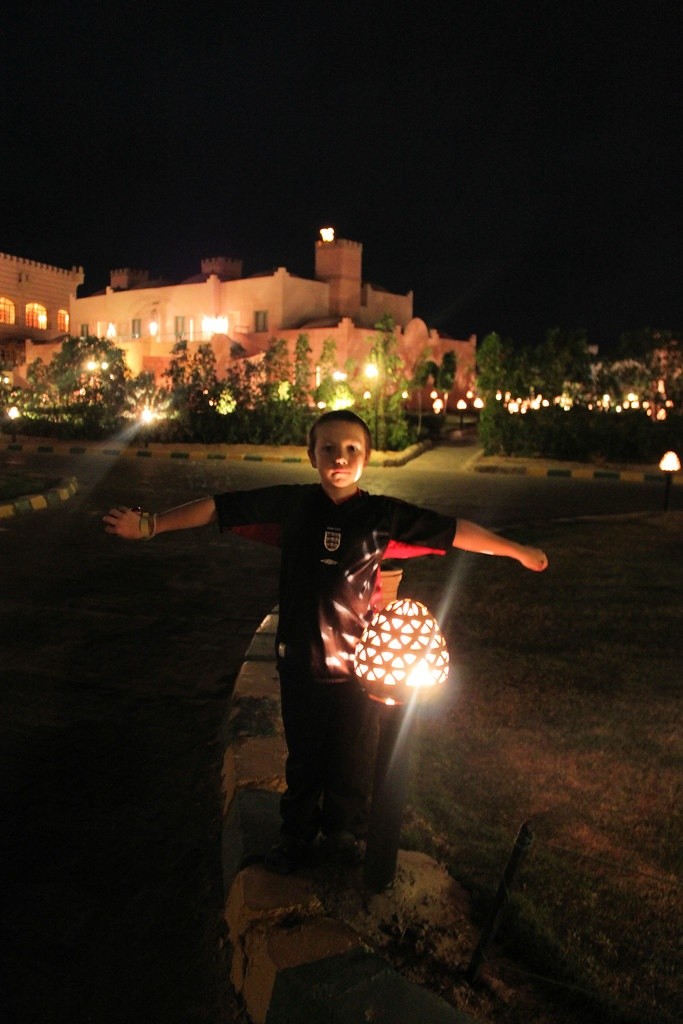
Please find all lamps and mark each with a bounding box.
[354,598,452,709]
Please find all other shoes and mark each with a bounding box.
[270,826,314,871]
[327,830,363,869]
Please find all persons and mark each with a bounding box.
[102,410,548,890]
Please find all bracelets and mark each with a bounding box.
[140,512,157,542]
